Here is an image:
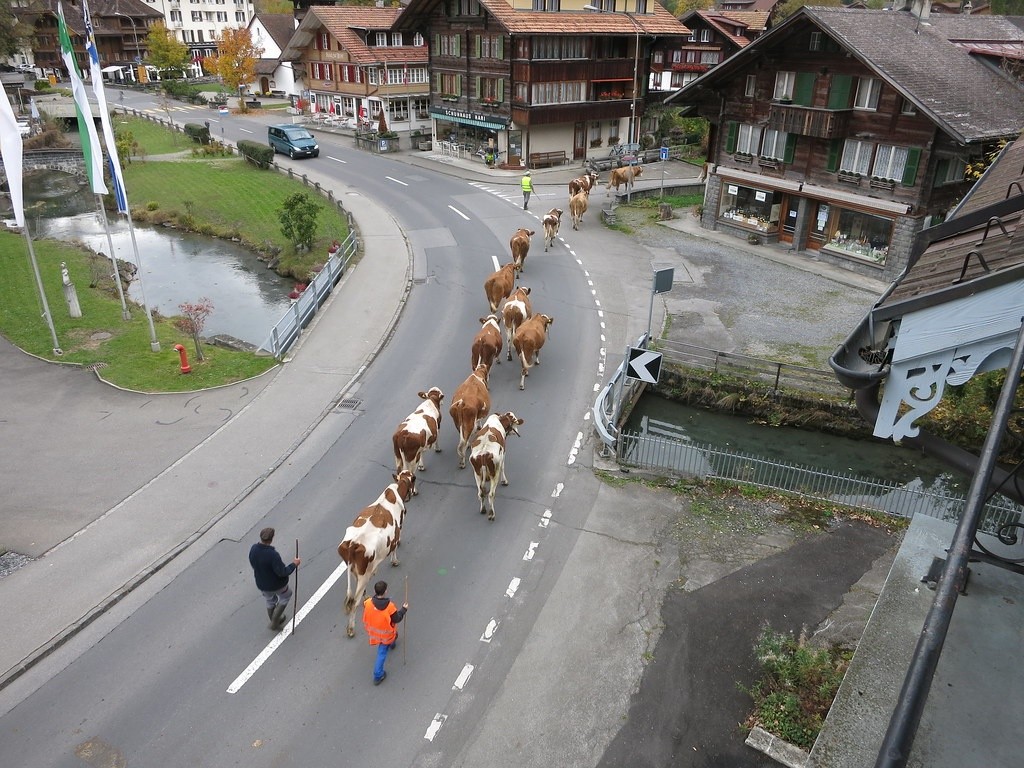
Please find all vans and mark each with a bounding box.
[268,123,319,160]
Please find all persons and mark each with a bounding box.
[364,580,408,688]
[520,171,534,211]
[250,529,301,632]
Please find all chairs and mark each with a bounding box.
[529,150,570,170]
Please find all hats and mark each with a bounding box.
[260,528,275,542]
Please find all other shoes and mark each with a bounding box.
[374,670,386,686]
[391,631,398,649]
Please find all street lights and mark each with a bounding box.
[583,4,639,204]
[115,12,141,65]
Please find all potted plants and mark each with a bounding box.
[440,94,450,101]
[758,156,780,170]
[448,95,458,102]
[780,94,794,105]
[837,169,862,185]
[869,177,896,191]
[734,151,753,164]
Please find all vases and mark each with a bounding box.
[480,102,499,107]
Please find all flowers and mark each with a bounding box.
[511,95,524,106]
[671,62,709,72]
[483,96,498,104]
[598,91,625,100]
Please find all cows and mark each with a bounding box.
[568,170,600,199]
[449,363,490,469]
[509,228,535,280]
[469,411,525,521]
[512,313,554,391]
[470,314,502,382]
[568,188,589,231]
[336,470,417,638]
[484,262,522,314]
[501,286,531,361]
[605,165,643,197]
[540,207,563,252]
[392,386,445,497]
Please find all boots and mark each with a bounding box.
[267,605,286,624]
[268,602,287,632]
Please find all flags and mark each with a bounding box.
[0,80,26,229]
[83,5,134,214]
[403,63,408,84]
[56,3,111,195]
[383,62,388,83]
